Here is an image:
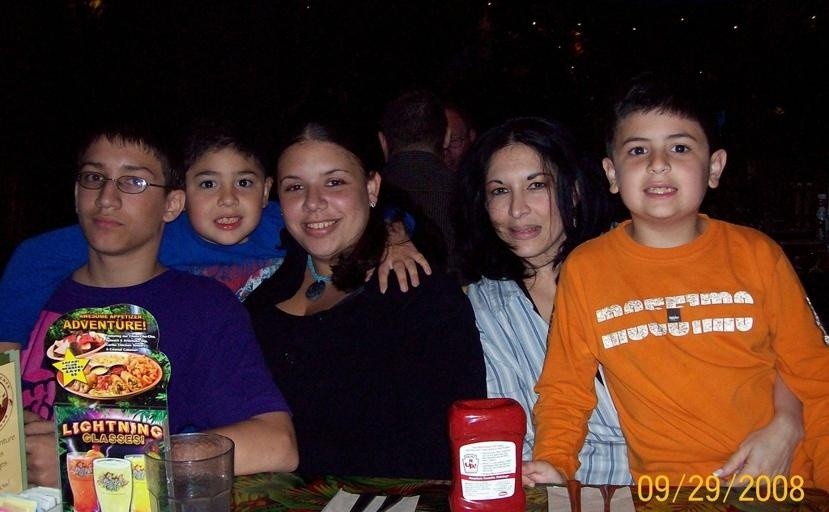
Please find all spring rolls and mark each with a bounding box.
[111,370,143,394]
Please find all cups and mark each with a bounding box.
[124,453,160,512]
[144,432,237,512]
[92,456,133,512]
[66,450,105,512]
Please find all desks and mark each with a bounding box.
[45,471,758,510]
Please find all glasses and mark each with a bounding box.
[450,136,466,148]
[74,171,170,194]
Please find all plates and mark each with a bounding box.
[56,350,164,402]
[46,331,109,361]
